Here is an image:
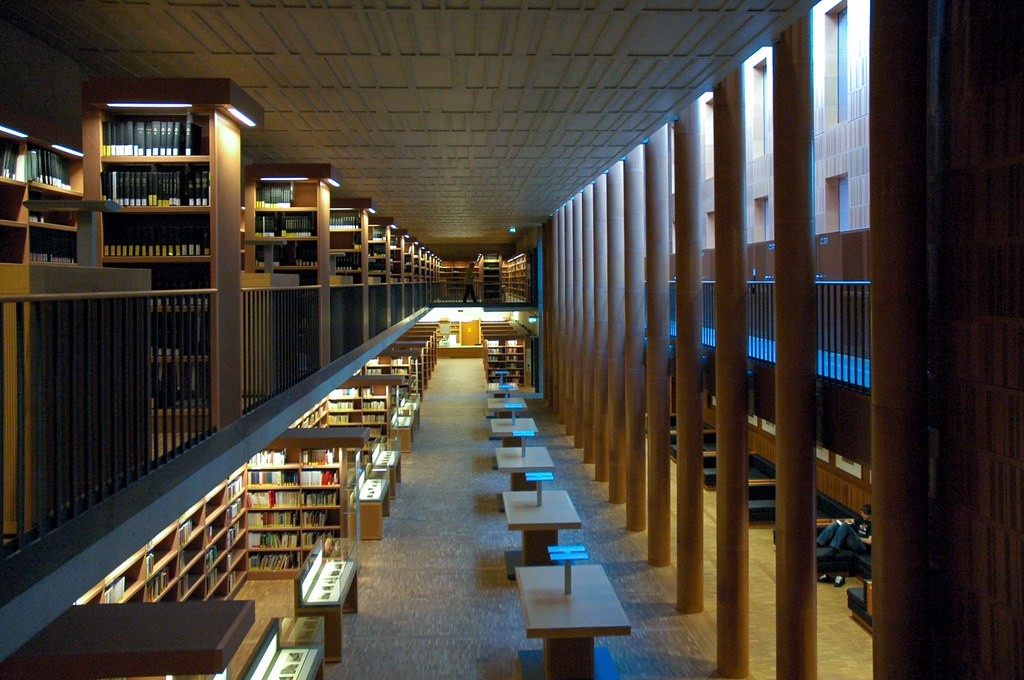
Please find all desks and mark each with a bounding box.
[488,382,519,396]
[514,564,633,680]
[502,488,582,581]
[488,398,528,420]
[495,446,555,513]
[492,417,539,470]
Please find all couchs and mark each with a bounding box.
[668,415,871,631]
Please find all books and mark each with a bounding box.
[0,145,77,263]
[247,448,339,569]
[368,356,407,407]
[390,236,398,274]
[297,387,385,435]
[368,243,386,272]
[487,340,518,383]
[484,263,500,299]
[373,229,382,241]
[330,216,359,229]
[103,121,211,408]
[336,251,362,270]
[256,185,317,286]
[104,476,243,602]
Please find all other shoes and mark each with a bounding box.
[818,574,828,583]
[834,576,846,587]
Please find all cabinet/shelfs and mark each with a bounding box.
[479,320,531,388]
[1,75,441,449]
[446,253,532,304]
[69,449,348,600]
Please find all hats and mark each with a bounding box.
[862,504,872,515]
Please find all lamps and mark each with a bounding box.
[500,384,514,402]
[512,430,535,456]
[505,402,523,426]
[526,472,554,508]
[494,370,508,386]
[548,544,588,595]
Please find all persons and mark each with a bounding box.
[463,263,477,302]
[287,654,298,661]
[817,504,872,556]
